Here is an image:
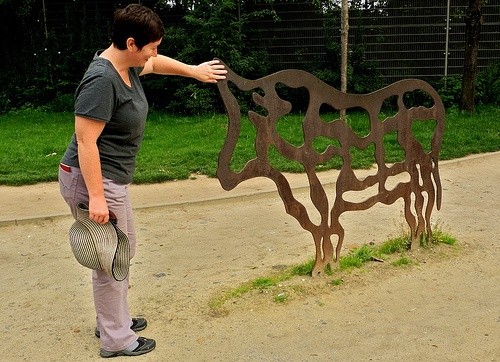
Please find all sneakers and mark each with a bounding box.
[95,316,148,338]
[98,336,156,357]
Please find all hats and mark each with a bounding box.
[69,201,132,282]
